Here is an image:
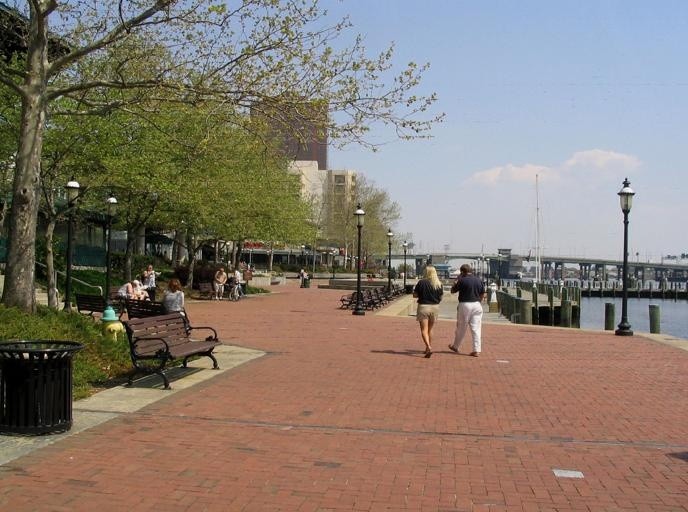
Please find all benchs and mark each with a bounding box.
[123,311,222,390]
[198,283,216,299]
[340,284,407,311]
[109,286,123,304]
[125,298,187,320]
[75,294,123,321]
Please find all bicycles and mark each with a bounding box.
[229,285,241,301]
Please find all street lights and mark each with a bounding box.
[352,203,365,316]
[103,193,120,310]
[470,257,480,279]
[301,244,305,270]
[615,177,635,337]
[402,240,408,295]
[332,249,337,278]
[63,175,84,313]
[426,253,429,266]
[386,227,394,301]
[486,257,489,287]
[225,241,229,270]
[497,251,502,290]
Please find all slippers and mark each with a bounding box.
[425,352,431,358]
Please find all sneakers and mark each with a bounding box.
[449,344,458,352]
[470,352,480,356]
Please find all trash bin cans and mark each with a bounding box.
[147,287,156,302]
[1,341,84,436]
[304,278,310,288]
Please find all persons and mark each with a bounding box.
[116,264,162,303]
[299,269,308,286]
[367,275,373,281]
[448,264,485,357]
[163,278,185,316]
[233,268,244,297]
[212,267,227,300]
[412,266,444,358]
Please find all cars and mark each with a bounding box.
[449,269,461,279]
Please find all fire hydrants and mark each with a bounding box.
[100,306,124,343]
